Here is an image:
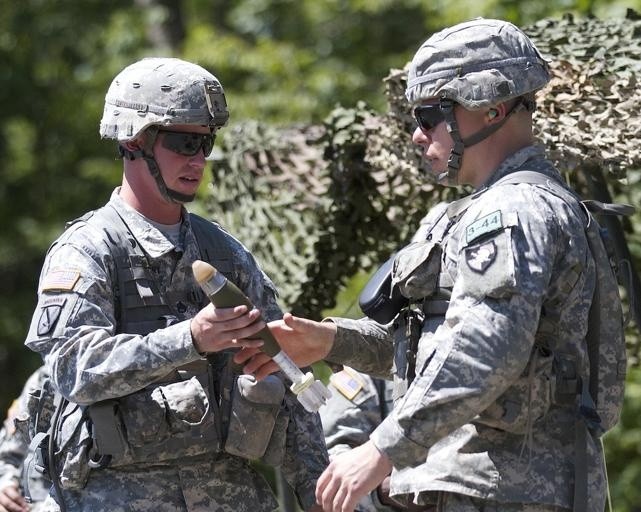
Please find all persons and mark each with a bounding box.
[233,17,623,512]
[0,360,53,512]
[308,368,407,512]
[21,57,332,512]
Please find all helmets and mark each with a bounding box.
[99,56,230,142]
[405,19,550,113]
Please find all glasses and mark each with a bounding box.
[413,102,460,131]
[148,127,216,155]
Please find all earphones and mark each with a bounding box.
[489,110,498,121]
